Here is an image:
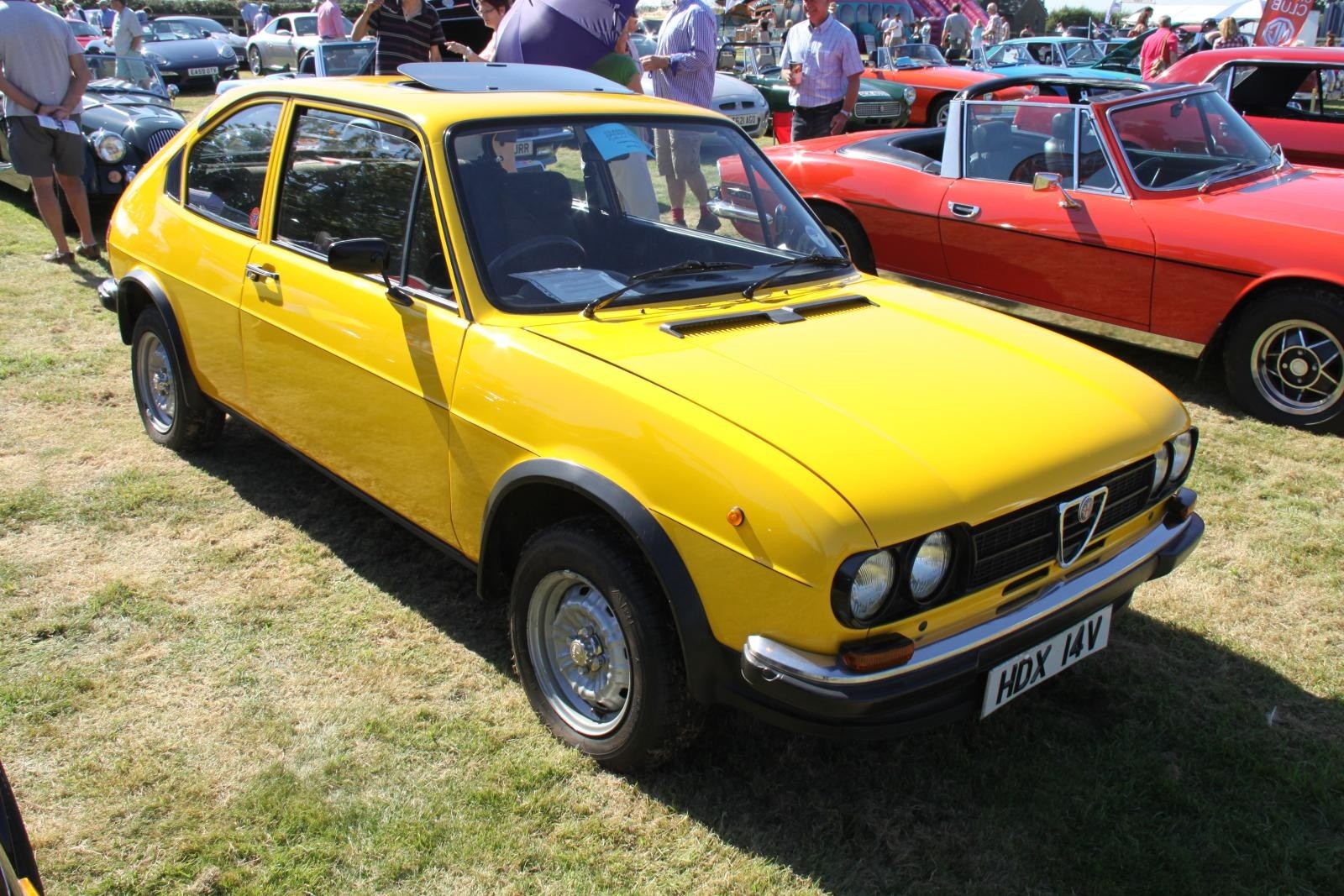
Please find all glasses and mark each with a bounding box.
[478,7,495,16]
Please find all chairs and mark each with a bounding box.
[1028,49,1040,63]
[752,55,775,72]
[868,50,885,68]
[1045,51,1057,66]
[201,155,589,275]
[946,48,962,65]
[773,111,793,144]
[972,110,1102,183]
[718,55,737,72]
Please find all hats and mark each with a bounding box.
[95,0,108,6]
[259,3,269,13]
[67,0,74,6]
[920,17,927,22]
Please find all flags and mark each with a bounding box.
[1253,0,1314,47]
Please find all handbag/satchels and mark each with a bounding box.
[1150,32,1169,78]
[1127,29,1141,38]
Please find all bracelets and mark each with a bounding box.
[35,102,42,114]
[463,47,472,59]
[841,109,852,117]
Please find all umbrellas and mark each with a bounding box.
[493,0,639,73]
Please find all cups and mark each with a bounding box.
[788,60,803,85]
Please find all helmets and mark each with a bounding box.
[1199,18,1216,33]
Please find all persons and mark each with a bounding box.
[444,0,722,233]
[1194,18,1250,90]
[311,0,345,37]
[238,0,274,35]
[1128,7,1178,81]
[0,0,100,262]
[352,0,446,152]
[941,4,1064,64]
[1325,32,1333,48]
[878,14,932,57]
[39,0,152,89]
[757,0,866,142]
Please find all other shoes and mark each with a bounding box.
[75,244,100,261]
[40,249,74,265]
[673,220,688,228]
[695,214,721,233]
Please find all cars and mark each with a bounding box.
[88,58,1206,783]
[703,80,1344,439]
[0,0,1344,241]
